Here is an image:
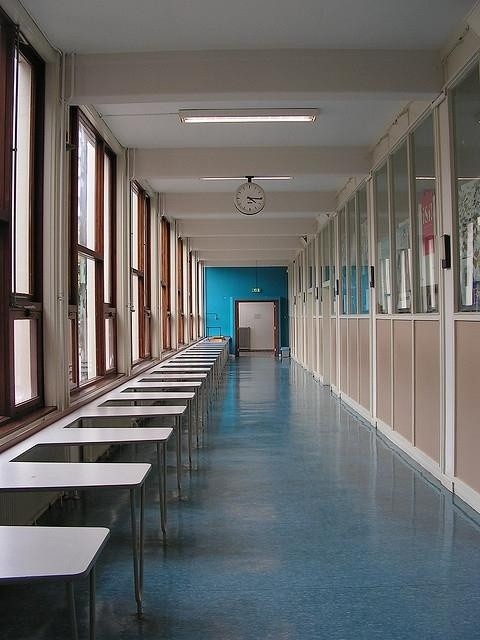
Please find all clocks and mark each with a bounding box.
[232,176,266,216]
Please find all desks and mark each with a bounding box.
[29,422,177,553]
[126,343,226,454]
[4,520,114,638]
[98,389,197,476]
[3,458,159,622]
[73,402,189,503]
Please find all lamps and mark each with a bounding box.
[199,176,295,181]
[178,107,322,125]
